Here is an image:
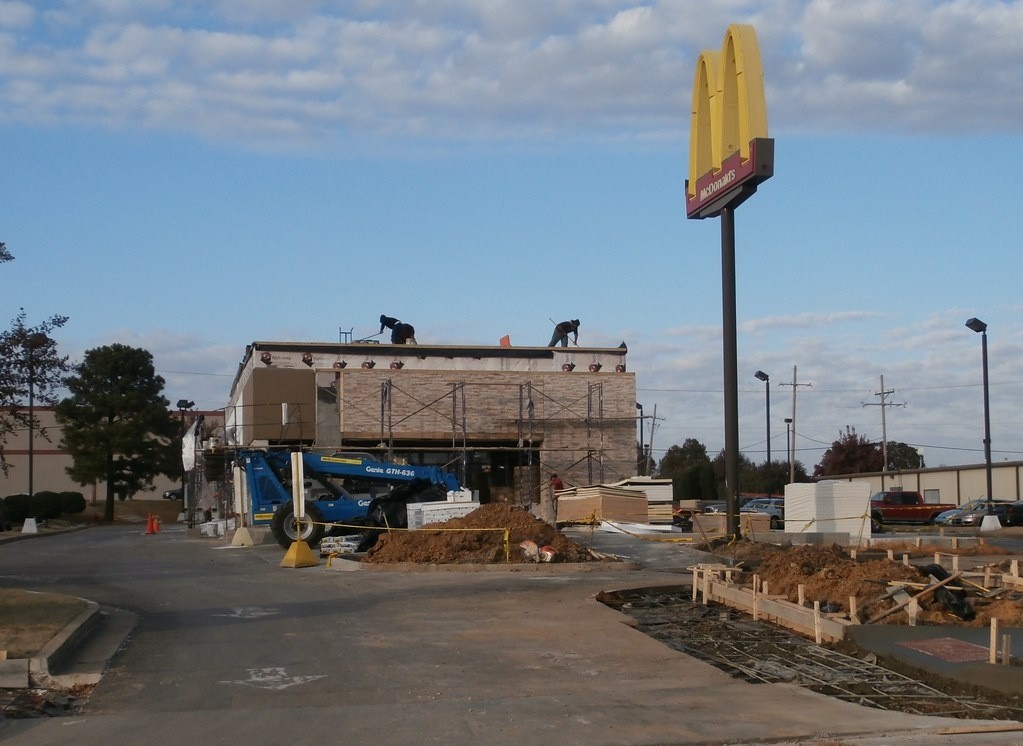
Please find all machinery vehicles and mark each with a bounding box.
[232,447,465,551]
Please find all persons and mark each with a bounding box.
[550,473,562,515]
[380,315,414,345]
[547,319,581,347]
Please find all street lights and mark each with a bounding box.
[22,332,49,535]
[964,317,992,501]
[177,399,195,486]
[753,370,771,499]
[636,403,643,475]
[784,417,792,483]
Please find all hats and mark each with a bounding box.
[571,319,580,326]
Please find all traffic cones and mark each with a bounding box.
[145,513,160,534]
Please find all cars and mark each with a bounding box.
[704,490,1023,532]
[162,487,183,501]
